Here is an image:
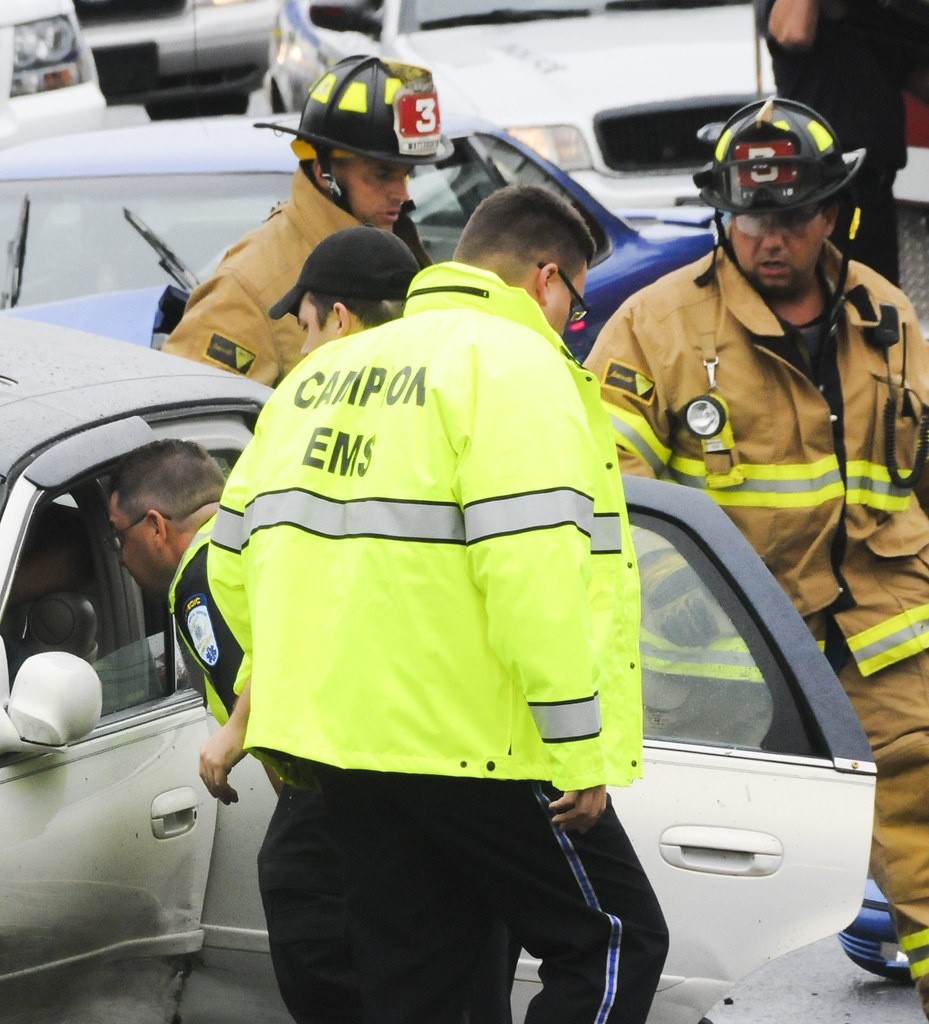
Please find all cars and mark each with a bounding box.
[0,316,883,1022]
[247,0,786,250]
[0,113,717,503]
[4,0,271,114]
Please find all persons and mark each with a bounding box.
[102,0,929,1024]
[206,185,669,1024]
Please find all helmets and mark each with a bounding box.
[255,54,455,164]
[693,96,867,216]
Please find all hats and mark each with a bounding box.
[269,222,420,321]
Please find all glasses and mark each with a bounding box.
[103,512,172,552]
[537,259,590,322]
[732,201,831,237]
